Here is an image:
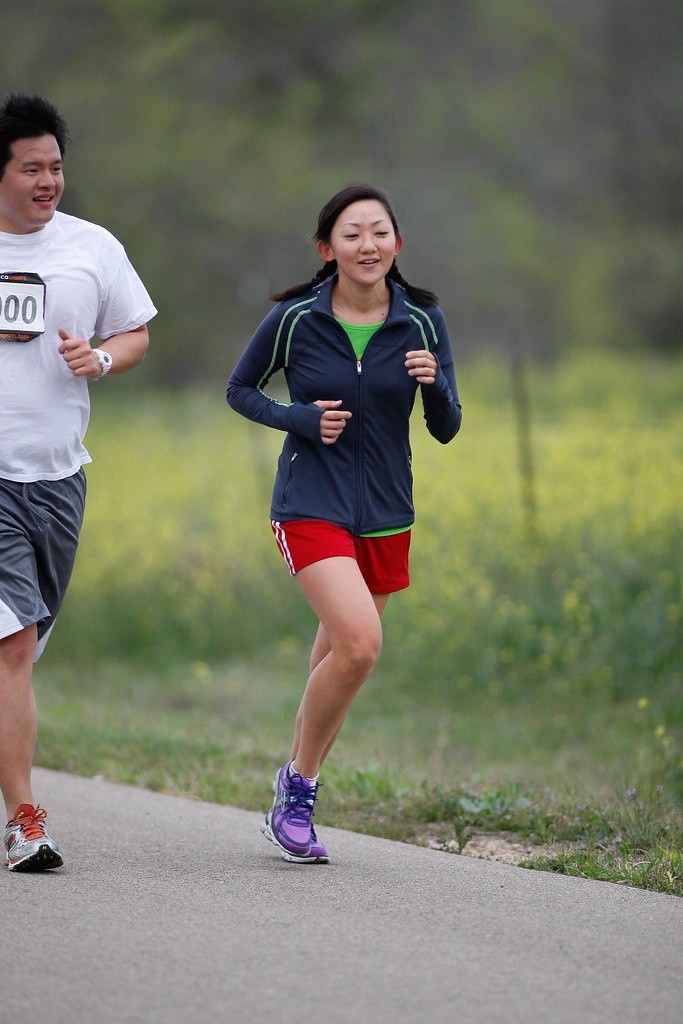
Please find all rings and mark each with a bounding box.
[431,368,435,376]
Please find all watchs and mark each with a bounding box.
[85,348,113,382]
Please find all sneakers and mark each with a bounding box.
[260,808,330,863]
[3,804,64,872]
[268,756,324,856]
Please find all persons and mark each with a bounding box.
[225,182,462,865]
[0,91,158,872]
